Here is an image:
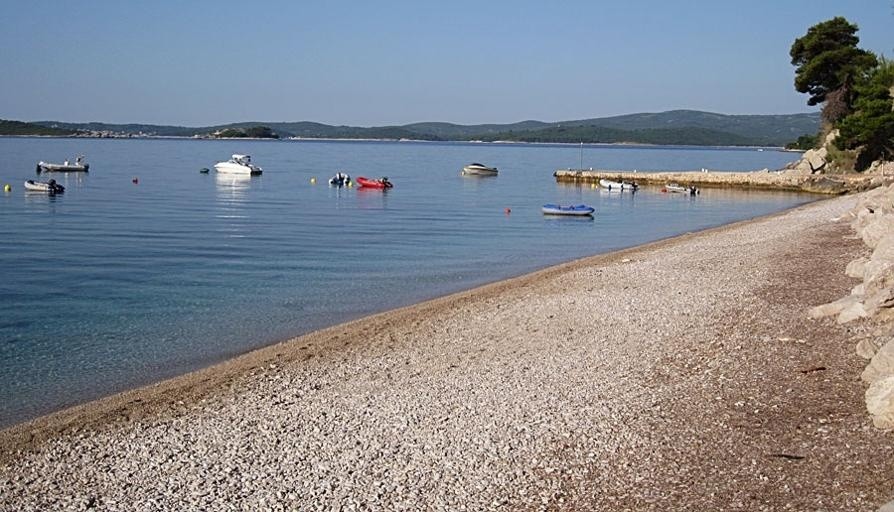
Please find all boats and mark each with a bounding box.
[464,163,497,177]
[666,182,685,192]
[25,181,64,194]
[357,176,393,189]
[329,171,351,187]
[40,161,89,172]
[542,204,594,216]
[215,155,262,176]
[600,180,639,190]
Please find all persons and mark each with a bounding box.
[64,159,70,166]
